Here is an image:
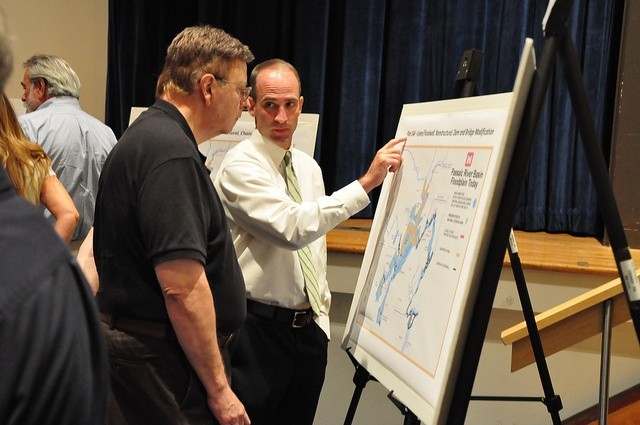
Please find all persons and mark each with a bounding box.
[214,58,407,425]
[0,92,81,250]
[17,54,119,260]
[92,27,257,425]
[1,169,110,425]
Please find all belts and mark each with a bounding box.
[95,311,237,349]
[246,299,315,329]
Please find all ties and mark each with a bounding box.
[282,153,320,317]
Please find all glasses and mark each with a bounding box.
[199,70,252,100]
